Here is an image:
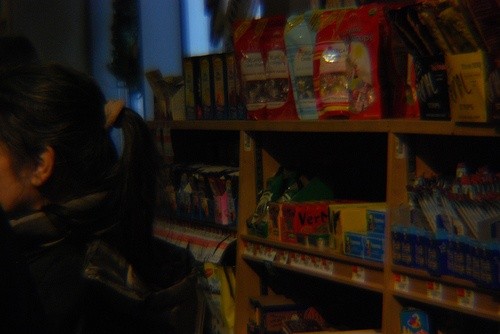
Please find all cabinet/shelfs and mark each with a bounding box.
[124,115,500,334]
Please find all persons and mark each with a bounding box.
[1,62,201,334]
